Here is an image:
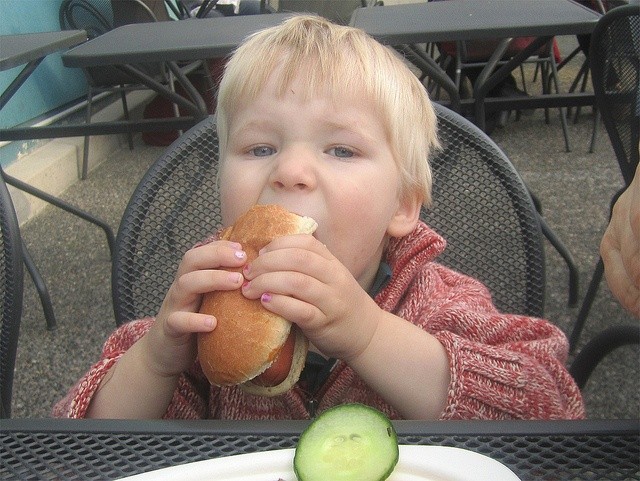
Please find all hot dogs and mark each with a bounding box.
[197,203,318,395]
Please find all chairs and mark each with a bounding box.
[58,0,205,180]
[432,38,577,155]
[0,173,25,417]
[111,0,217,150]
[567,3,640,354]
[109,92,545,344]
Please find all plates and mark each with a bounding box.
[117,445,519,476]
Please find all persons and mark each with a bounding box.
[53,13,587,419]
[598,143,640,319]
